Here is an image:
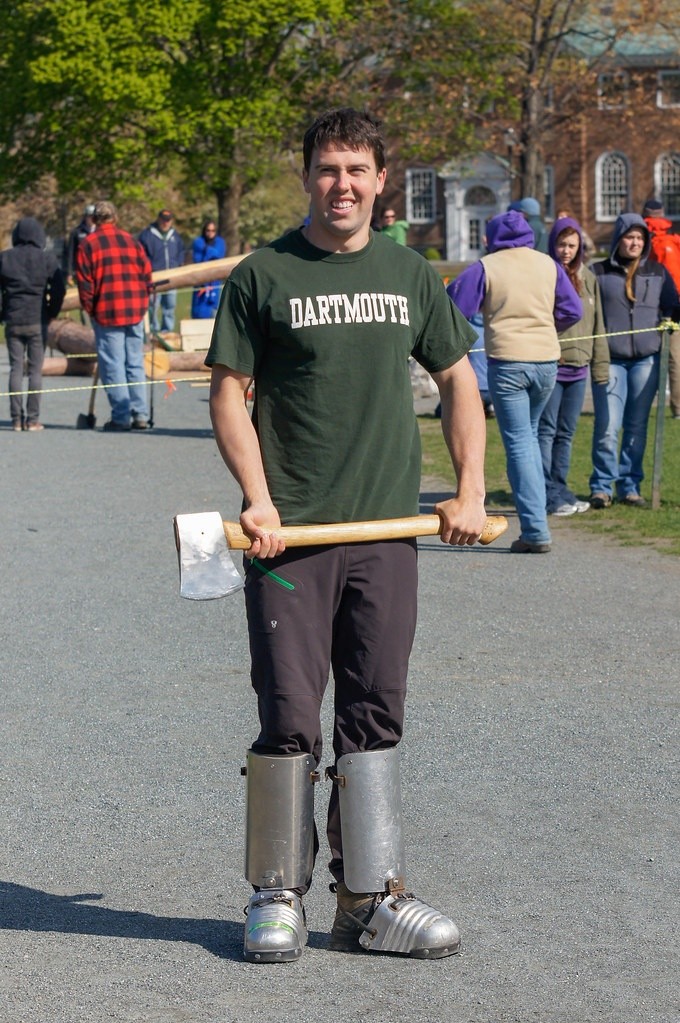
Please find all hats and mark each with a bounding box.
[641,200,663,219]
[158,209,175,220]
[506,202,523,214]
[87,205,96,215]
[517,198,542,216]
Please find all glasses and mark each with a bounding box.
[206,229,216,233]
[384,215,396,219]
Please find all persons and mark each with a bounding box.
[520,197,549,254]
[446,210,583,553]
[641,201,680,296]
[378,206,409,246]
[0,217,67,432]
[139,210,185,334]
[64,204,96,325]
[76,200,153,431]
[555,210,596,263]
[588,213,680,508]
[203,106,487,963]
[538,218,610,515]
[190,220,226,319]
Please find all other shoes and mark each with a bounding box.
[572,499,591,513]
[104,420,131,432]
[27,422,45,431]
[552,502,577,518]
[129,420,147,429]
[590,491,611,509]
[14,424,23,430]
[328,882,388,950]
[511,535,550,553]
[621,493,647,508]
